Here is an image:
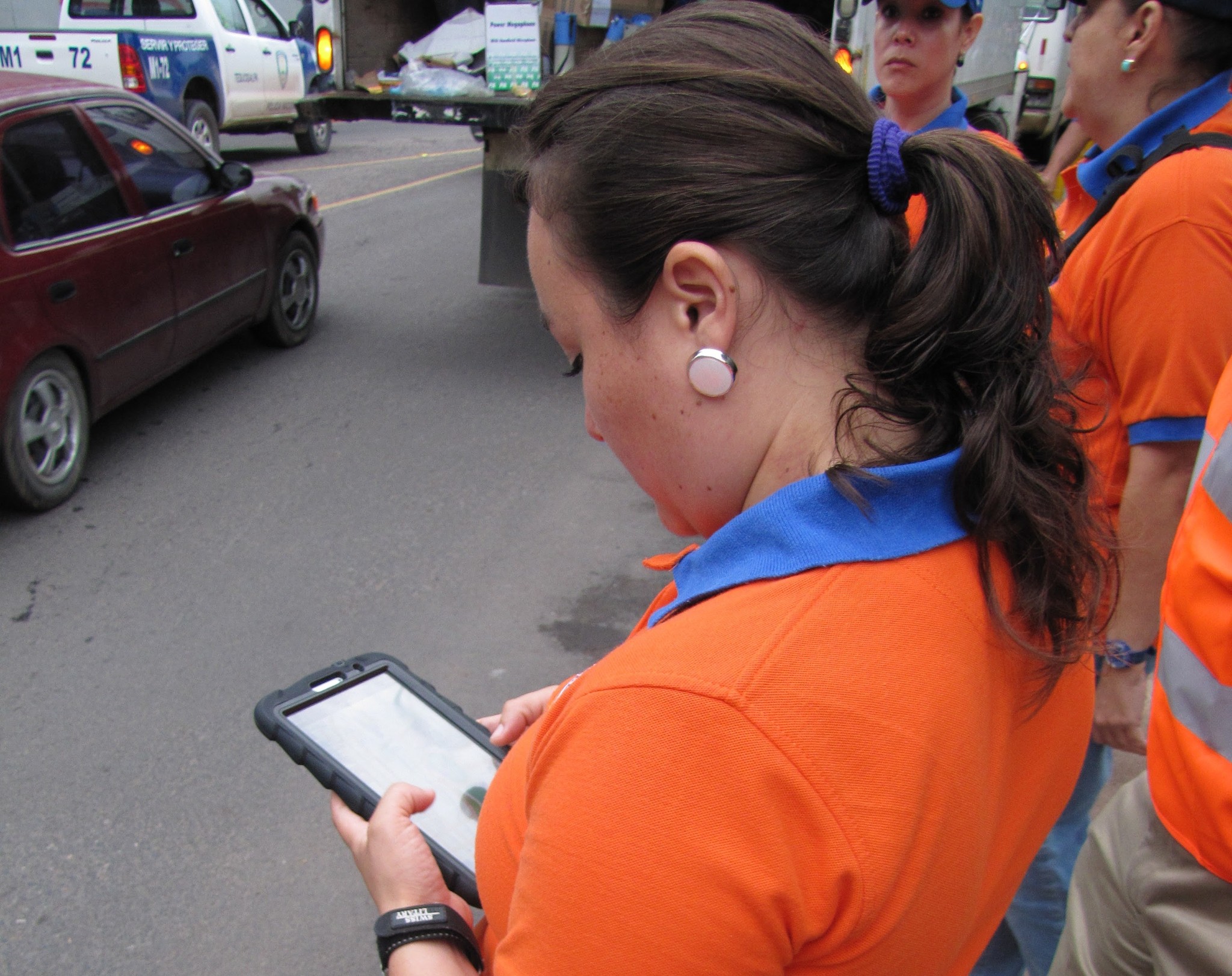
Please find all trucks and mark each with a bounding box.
[293,0,1081,289]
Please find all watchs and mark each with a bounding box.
[1101,639,1155,671]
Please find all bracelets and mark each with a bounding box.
[372,901,487,971]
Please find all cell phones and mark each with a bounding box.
[254,652,512,910]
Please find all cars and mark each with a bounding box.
[1,70,324,519]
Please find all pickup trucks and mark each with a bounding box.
[0,1,333,158]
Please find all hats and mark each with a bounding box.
[859,0,983,19]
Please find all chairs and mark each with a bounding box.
[132,0,161,16]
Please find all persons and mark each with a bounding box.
[867,0,1026,254]
[970,0,1231,976]
[324,0,1123,976]
